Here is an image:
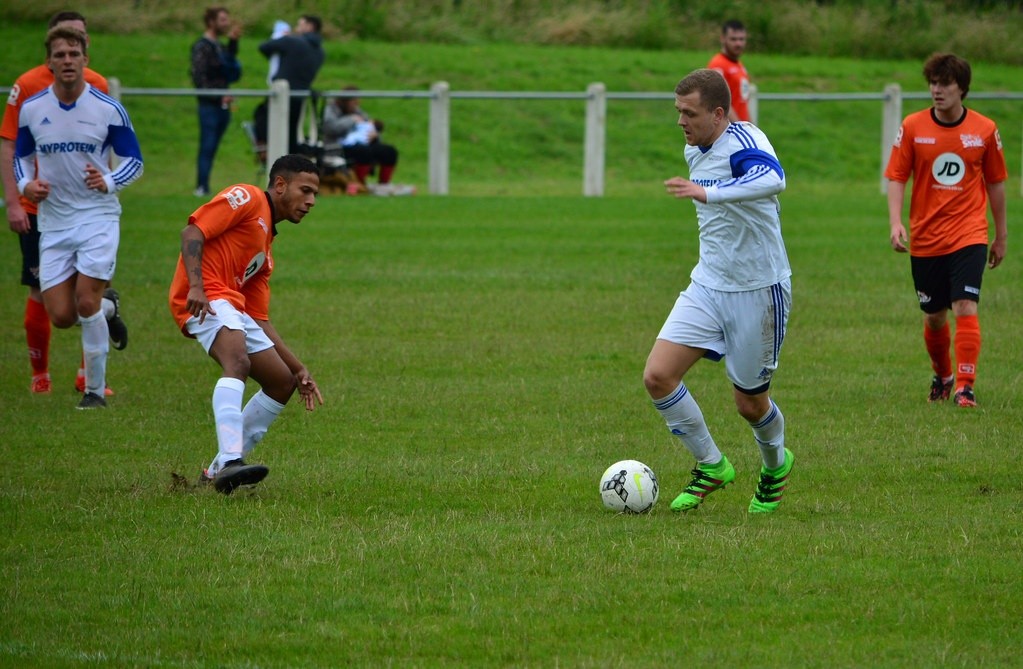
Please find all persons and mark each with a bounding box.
[13,23,143,409]
[187,7,241,198]
[645,69,795,512]
[707,19,758,126]
[0,8,112,396]
[250,16,325,168]
[319,85,399,197]
[883,54,1009,406]
[168,152,319,493]
[251,98,371,195]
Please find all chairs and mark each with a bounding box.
[242,121,369,193]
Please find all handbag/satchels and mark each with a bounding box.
[296,88,325,173]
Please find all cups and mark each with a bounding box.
[346,183,357,194]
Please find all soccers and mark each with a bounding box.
[599,460,659,514]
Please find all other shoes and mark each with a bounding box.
[372,182,397,196]
[193,187,207,197]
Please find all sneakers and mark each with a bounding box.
[954,385,977,407]
[925,375,954,403]
[668,454,736,513]
[76,392,108,409]
[198,468,214,486]
[74,372,114,396]
[213,458,268,494]
[103,288,128,351]
[32,377,53,394]
[748,446,795,515]
